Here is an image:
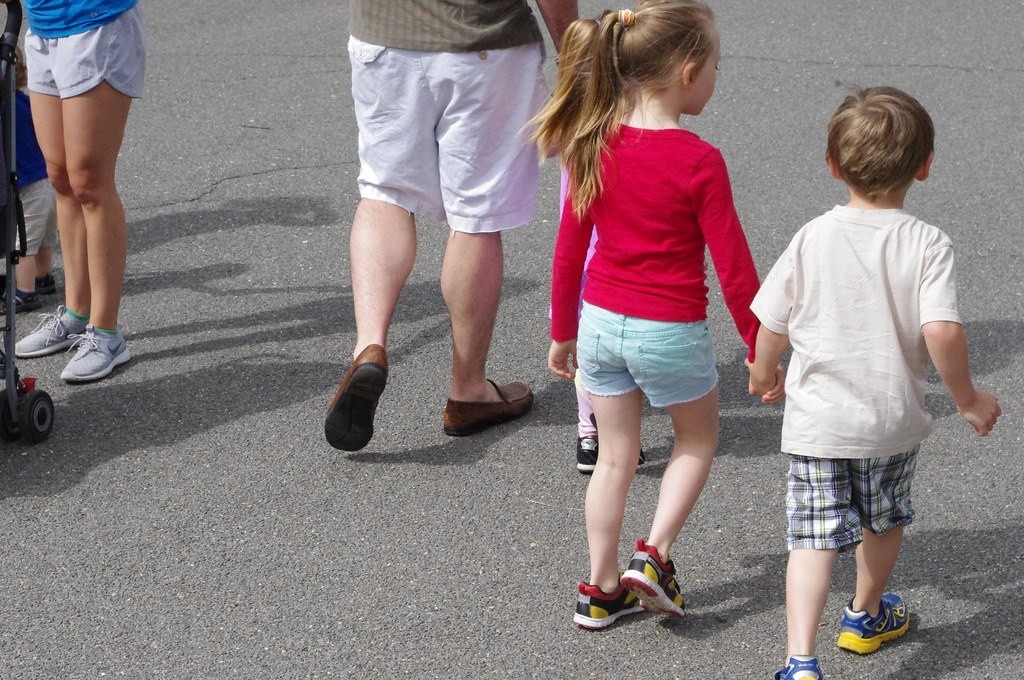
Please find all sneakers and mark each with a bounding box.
[573,571,646,630]
[60,324,130,381]
[837,593,909,655]
[15,305,86,358]
[620,538,685,618]
[774,655,824,680]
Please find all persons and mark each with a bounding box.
[25,0,144,380]
[514,0,784,628]
[325,0,579,452]
[0,45,58,316]
[749,84,1001,680]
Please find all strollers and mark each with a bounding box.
[0,0,56,444]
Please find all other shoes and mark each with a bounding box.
[444,379,534,436]
[576,436,599,472]
[590,412,645,465]
[325,344,388,452]
[36,272,56,294]
[0,289,42,315]
